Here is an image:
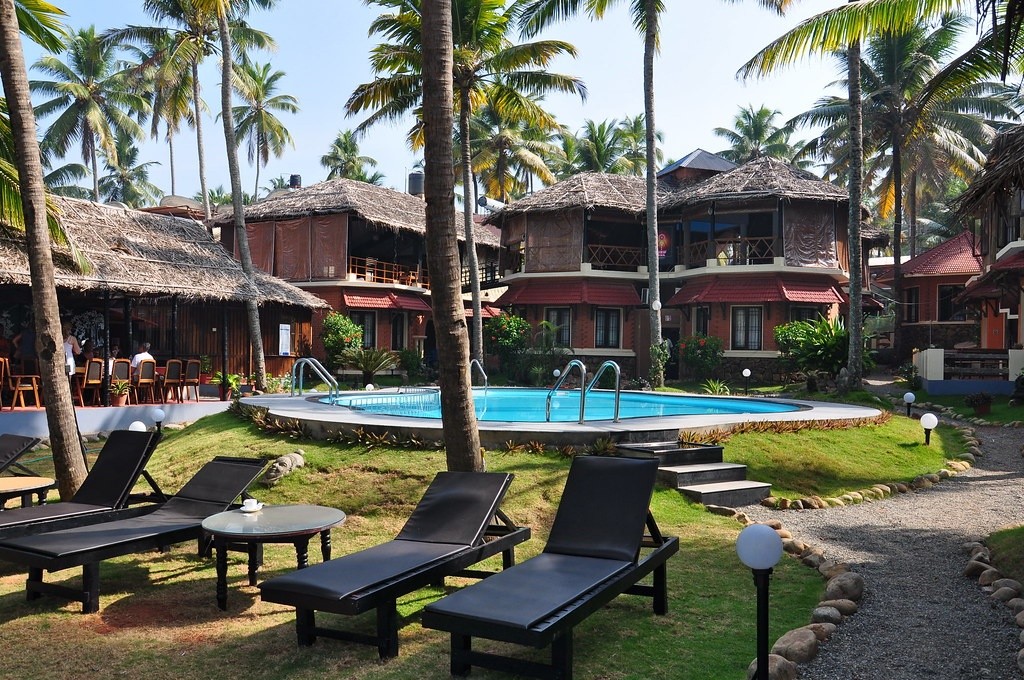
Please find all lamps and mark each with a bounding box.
[553,370,560,381]
[904,392,915,417]
[743,368,751,395]
[736,525,783,680]
[921,413,937,446]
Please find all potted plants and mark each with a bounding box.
[334,347,397,386]
[210,373,239,400]
[108,376,132,407]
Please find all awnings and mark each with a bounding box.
[489,280,885,320]
[950,249,1024,316]
[343,287,430,328]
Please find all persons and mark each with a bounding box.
[130,342,155,403]
[12,321,127,386]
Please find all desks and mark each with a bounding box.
[0,477,55,511]
[202,505,346,611]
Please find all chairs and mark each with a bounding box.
[259,471,531,664]
[0,429,172,531]
[0,455,269,614]
[0,433,40,478]
[423,454,680,680]
[0,356,202,410]
